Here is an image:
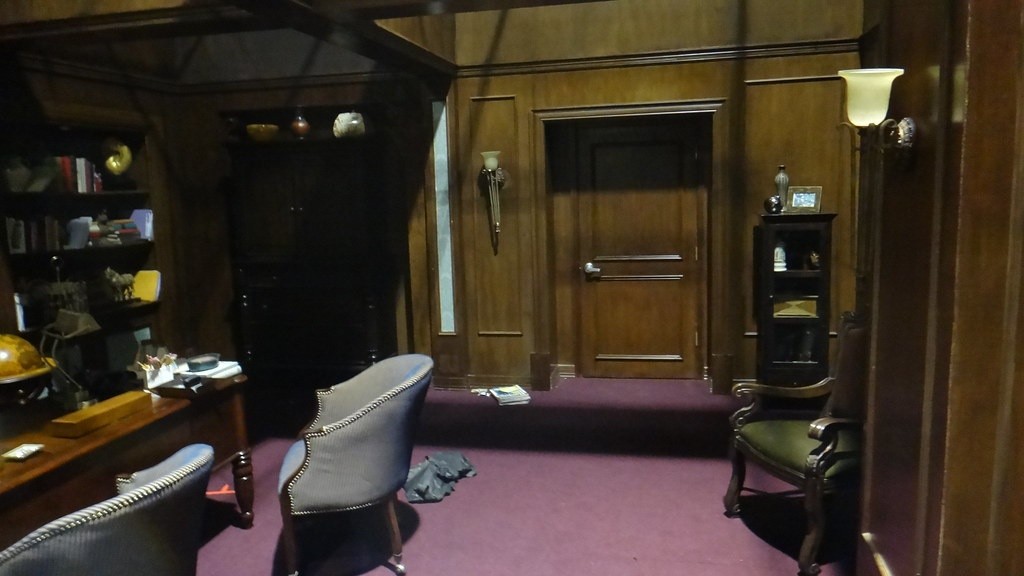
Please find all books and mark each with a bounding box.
[489,384,531,405]
[42,155,103,193]
[106,220,141,242]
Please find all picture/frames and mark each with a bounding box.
[786,186,822,214]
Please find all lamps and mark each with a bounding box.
[481,150,504,234]
[837,68,917,187]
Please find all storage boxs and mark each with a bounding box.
[773,295,818,317]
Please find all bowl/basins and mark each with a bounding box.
[186,352,221,372]
[246,124,278,140]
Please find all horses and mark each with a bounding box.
[103,266,136,302]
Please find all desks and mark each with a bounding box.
[0,372,248,551]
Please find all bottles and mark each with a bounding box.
[291,104,311,140]
[774,165,789,212]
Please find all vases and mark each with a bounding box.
[774,165,788,213]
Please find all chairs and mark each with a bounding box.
[276,354,433,576]
[723,301,875,576]
[0,443,215,576]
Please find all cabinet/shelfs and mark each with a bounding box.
[0,98,177,392]
[752,212,837,408]
